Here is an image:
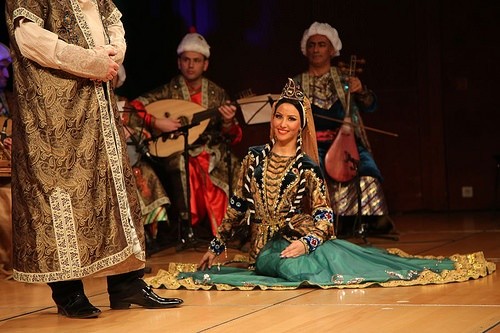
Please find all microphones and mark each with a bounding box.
[131,133,150,158]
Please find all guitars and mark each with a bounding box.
[128,99,234,159]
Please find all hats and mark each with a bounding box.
[176,25,210,58]
[301,21,342,57]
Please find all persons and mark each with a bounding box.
[279,21,394,238]
[197,96,415,282]
[113,63,171,241]
[0,41,12,277]
[4,0,184,319]
[129,31,244,251]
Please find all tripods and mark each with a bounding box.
[146,121,248,257]
[336,157,400,247]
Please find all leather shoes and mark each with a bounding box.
[109,286,184,309]
[58,292,102,318]
